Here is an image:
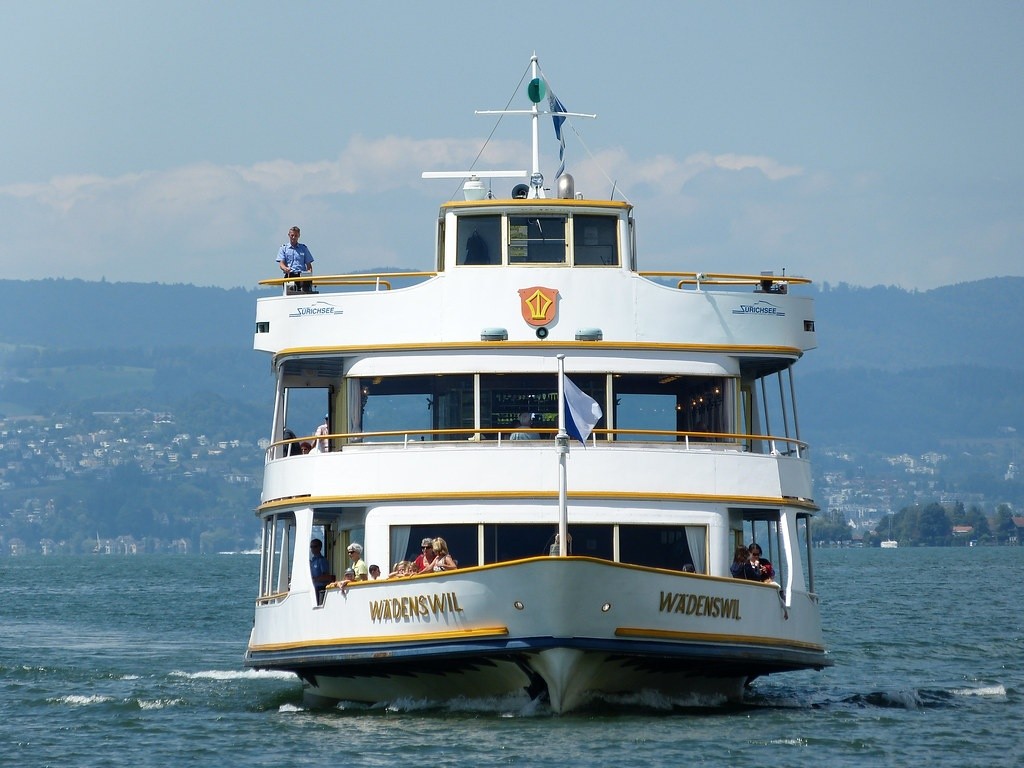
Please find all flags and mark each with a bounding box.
[541,77,567,181]
[563,375,603,449]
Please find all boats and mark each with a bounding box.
[880,539,898,549]
[241,50,837,715]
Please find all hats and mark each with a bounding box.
[344,568,354,575]
[324,414,329,420]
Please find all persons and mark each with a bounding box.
[310,537,458,606]
[510,412,541,441]
[281,414,329,457]
[275,227,315,295]
[730,542,776,583]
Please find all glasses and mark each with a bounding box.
[421,546,431,549]
[348,550,355,554]
[310,545,319,548]
[750,552,760,556]
